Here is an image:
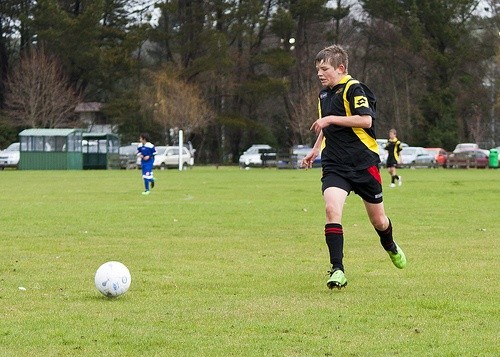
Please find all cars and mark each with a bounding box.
[110,143,146,169]
[238,144,278,169]
[0,141,51,170]
[377,139,423,169]
[412,148,449,170]
[453,143,480,156]
[443,151,489,171]
[62,139,111,157]
[288,144,323,169]
[137,144,193,171]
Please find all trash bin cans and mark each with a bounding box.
[489,149,498,167]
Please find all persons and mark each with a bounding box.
[385,128,403,186]
[137,135,155,192]
[302,47,405,288]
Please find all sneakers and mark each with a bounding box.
[142,190,150,194]
[389,183,396,187]
[398,176,401,186]
[327,266,347,287]
[380,237,406,269]
[150,181,154,188]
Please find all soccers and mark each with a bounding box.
[95,261,132,297]
